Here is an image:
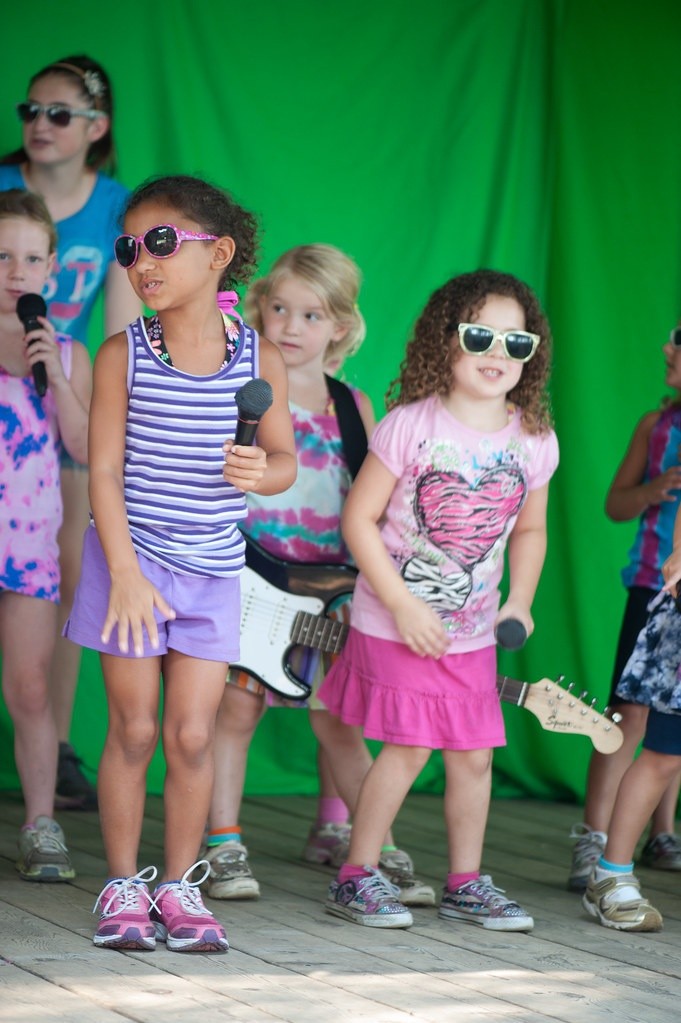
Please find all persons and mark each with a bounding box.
[567,320,681,930]
[1,185,96,883]
[1,52,144,810]
[316,268,559,929]
[205,245,434,907]
[60,175,298,953]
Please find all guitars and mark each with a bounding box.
[228,528,626,753]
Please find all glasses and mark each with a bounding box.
[447,322,541,362]
[671,326,681,349]
[19,103,109,126]
[115,224,220,269]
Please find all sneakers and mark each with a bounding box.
[379,851,435,906]
[15,815,75,882]
[148,860,229,952]
[582,875,663,932]
[570,823,608,892]
[438,875,534,932]
[301,823,351,867]
[55,743,98,811]
[641,832,681,871]
[326,864,414,929]
[199,839,260,899]
[93,866,161,951]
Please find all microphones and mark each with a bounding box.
[16,293,48,397]
[233,378,274,448]
[496,620,527,648]
